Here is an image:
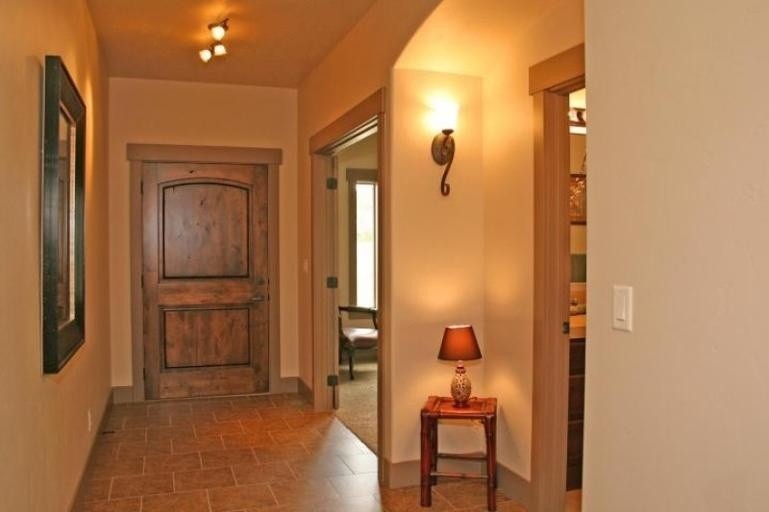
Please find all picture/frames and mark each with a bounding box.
[42,53,86,373]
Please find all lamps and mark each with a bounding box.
[427,88,460,196]
[437,325,482,410]
[200,10,229,65]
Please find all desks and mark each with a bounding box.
[419,395,498,511]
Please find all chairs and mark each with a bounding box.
[338,305,379,380]
